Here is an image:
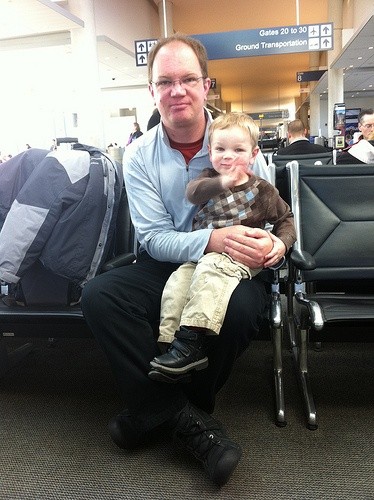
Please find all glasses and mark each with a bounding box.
[150,75,204,89]
[360,124,374,129]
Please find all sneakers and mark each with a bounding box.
[147,325,208,384]
[109,409,167,449]
[171,400,242,487]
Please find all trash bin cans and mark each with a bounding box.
[314,137,324,146]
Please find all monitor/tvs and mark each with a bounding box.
[333,107,361,130]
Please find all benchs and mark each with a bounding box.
[0,163,284,424]
[285,159,374,428]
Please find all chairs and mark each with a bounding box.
[264,149,336,165]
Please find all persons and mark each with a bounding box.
[127,123,143,144]
[148,111,295,381]
[80,33,274,485]
[276,119,329,155]
[341,108,374,153]
